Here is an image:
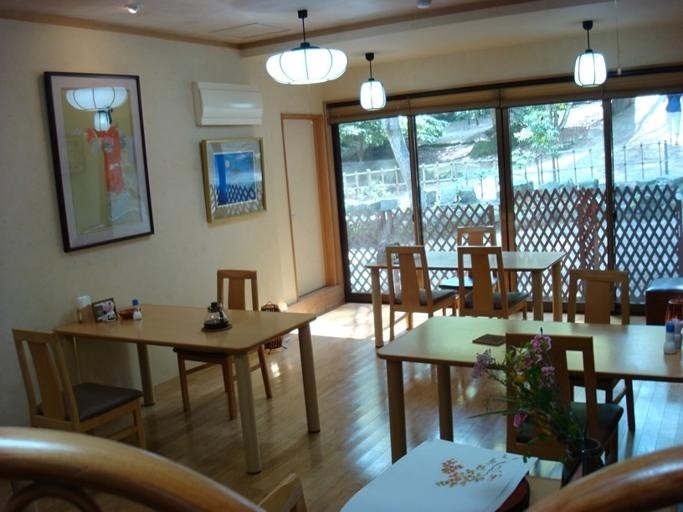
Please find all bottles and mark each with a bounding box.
[663,321,677,355]
[133,298,143,321]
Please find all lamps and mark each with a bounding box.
[64,86,129,133]
[357,52,386,113]
[264,7,349,87]
[572,18,606,90]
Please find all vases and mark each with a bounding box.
[562,438,605,486]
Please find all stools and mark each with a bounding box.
[645,277,682,326]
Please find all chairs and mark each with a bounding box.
[11,328,144,450]
[457,246,529,319]
[173,270,272,412]
[384,245,458,339]
[439,229,497,316]
[567,270,635,431]
[506,334,624,486]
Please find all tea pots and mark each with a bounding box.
[203,302,230,330]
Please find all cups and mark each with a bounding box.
[76,295,92,324]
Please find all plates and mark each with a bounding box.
[118,308,134,319]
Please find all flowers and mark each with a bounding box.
[471,333,575,440]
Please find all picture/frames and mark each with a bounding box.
[198,133,268,228]
[41,69,156,254]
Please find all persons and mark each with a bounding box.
[86,111,139,221]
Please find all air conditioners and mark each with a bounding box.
[191,81,264,128]
